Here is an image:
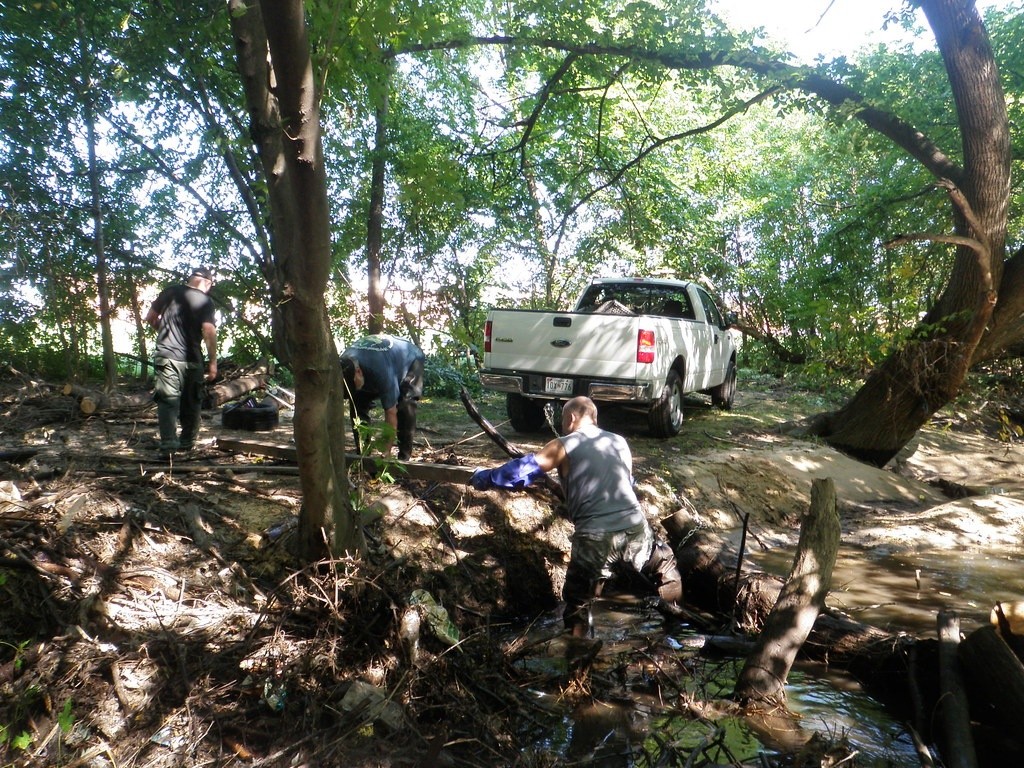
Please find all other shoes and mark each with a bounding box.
[158,449,175,459]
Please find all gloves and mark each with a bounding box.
[467,452,545,491]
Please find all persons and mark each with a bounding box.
[469,395,683,639]
[145,266,223,453]
[338,332,426,461]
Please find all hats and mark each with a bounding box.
[193,268,212,281]
[339,357,356,399]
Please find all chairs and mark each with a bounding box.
[663,300,683,318]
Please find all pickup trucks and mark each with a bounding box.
[480,278,738,436]
